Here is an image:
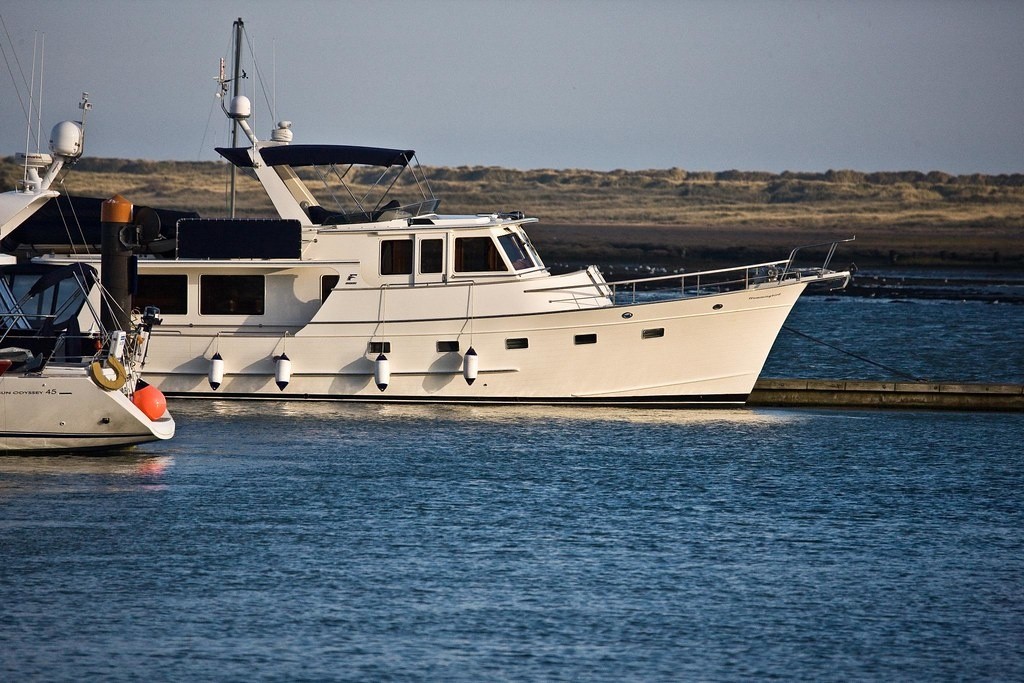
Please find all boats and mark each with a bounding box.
[0,27,179,457]
[0,14,858,405]
